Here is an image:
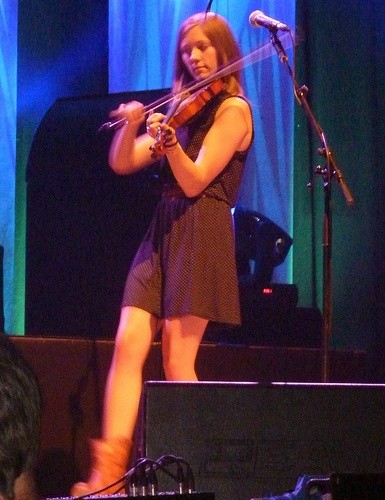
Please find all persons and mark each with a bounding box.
[71,12,254,499]
[0,330,43,500]
[251,474,331,500]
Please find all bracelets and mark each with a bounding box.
[163,138,178,149]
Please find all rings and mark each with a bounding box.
[146,127,149,129]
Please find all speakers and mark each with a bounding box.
[140,380,385,500]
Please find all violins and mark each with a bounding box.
[149,77,224,156]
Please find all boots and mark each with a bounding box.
[71,437,133,495]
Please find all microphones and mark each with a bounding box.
[250,11,289,32]
